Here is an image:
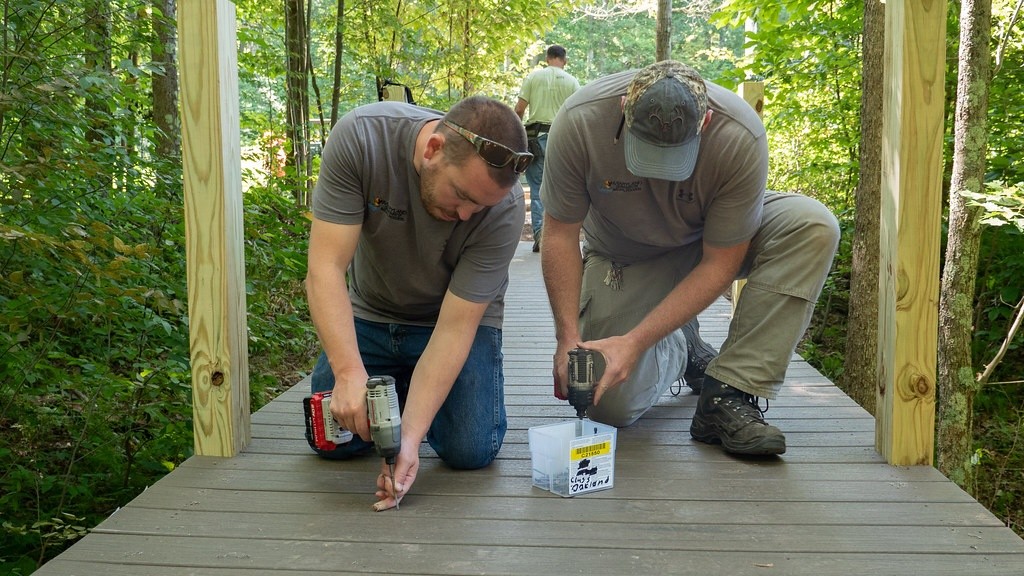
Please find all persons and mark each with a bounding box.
[305,97,536,510]
[539,58,838,457]
[514,46,582,250]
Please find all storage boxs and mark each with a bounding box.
[528,418,617,500]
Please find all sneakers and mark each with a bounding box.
[690,374,786,455]
[670,314,720,395]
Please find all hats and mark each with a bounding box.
[623,60,707,181]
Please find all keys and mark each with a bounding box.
[604,264,625,291]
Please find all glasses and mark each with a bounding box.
[443,120,534,173]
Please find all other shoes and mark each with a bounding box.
[532,231,542,251]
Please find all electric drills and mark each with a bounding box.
[303,376,402,510]
[567,349,598,420]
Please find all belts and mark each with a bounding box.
[527,122,550,133]
